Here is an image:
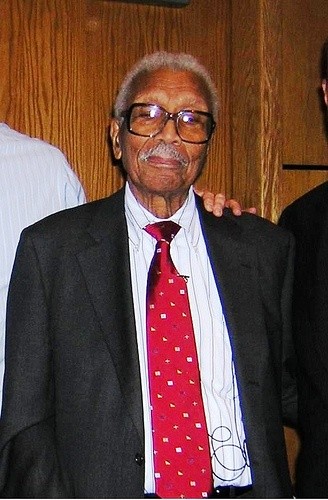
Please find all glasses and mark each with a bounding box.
[119,102,215,143]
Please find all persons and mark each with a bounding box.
[192,43,328,500]
[0,122,87,402]
[2,51,304,500]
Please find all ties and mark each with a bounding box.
[142,221,213,500]
